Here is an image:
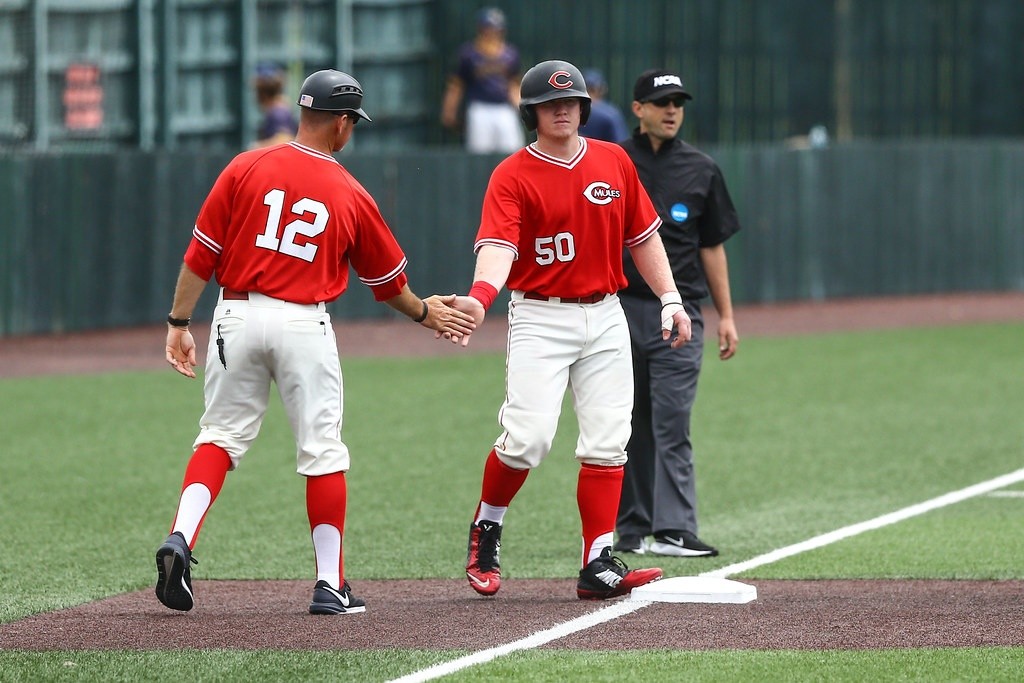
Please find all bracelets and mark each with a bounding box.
[167,313,190,327]
[413,300,428,322]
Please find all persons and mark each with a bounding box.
[578,67,631,145]
[615,72,742,558]
[443,9,524,155]
[435,60,692,599]
[156,70,477,615]
[248,75,297,147]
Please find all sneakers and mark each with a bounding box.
[613,535,650,556]
[155,531,199,612]
[308,578,366,614]
[649,530,720,559]
[576,545,664,600]
[466,519,504,596]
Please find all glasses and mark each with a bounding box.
[332,111,360,125]
[653,96,684,108]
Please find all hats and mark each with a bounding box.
[634,69,693,103]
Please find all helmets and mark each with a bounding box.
[296,69,374,123]
[519,60,592,131]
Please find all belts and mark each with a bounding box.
[223,289,248,301]
[524,293,616,305]
